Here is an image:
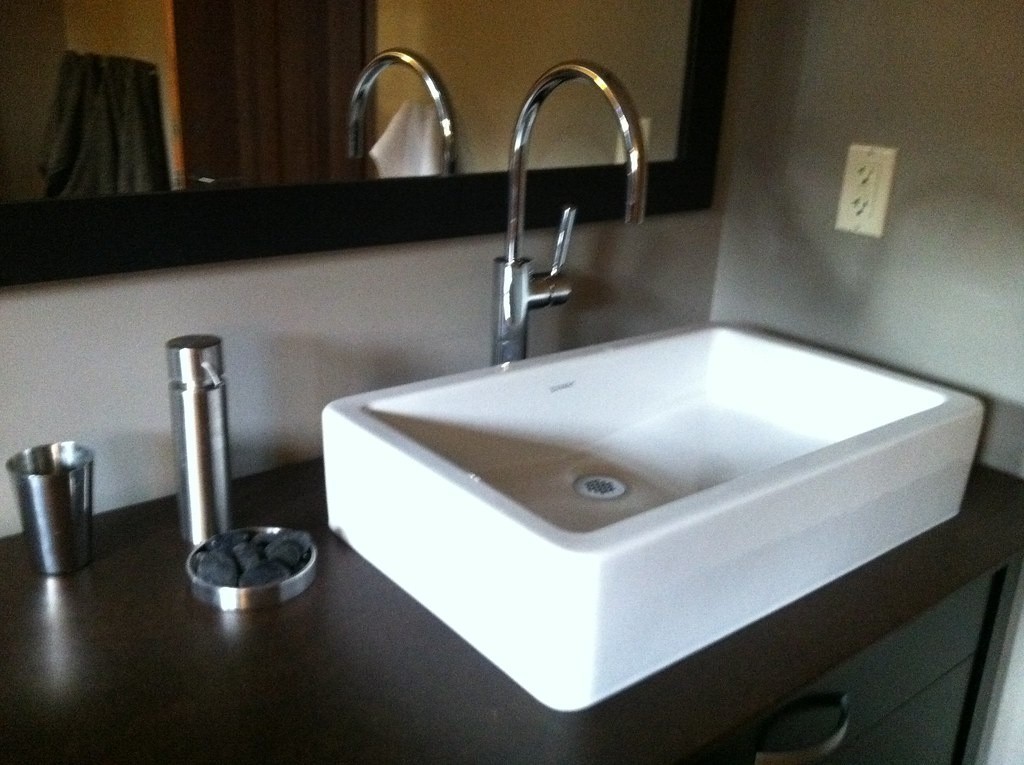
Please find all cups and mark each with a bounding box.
[4,441,94,575]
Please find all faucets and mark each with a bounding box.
[346,46,461,180]
[165,333,236,546]
[488,59,647,368]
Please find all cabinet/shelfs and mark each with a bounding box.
[671,555,1024,765]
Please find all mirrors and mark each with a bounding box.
[0,0,738,290]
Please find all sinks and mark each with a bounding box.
[320,319,989,713]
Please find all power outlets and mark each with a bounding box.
[614,115,651,164]
[832,144,899,240]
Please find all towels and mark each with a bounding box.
[366,98,452,180]
[31,47,175,200]
